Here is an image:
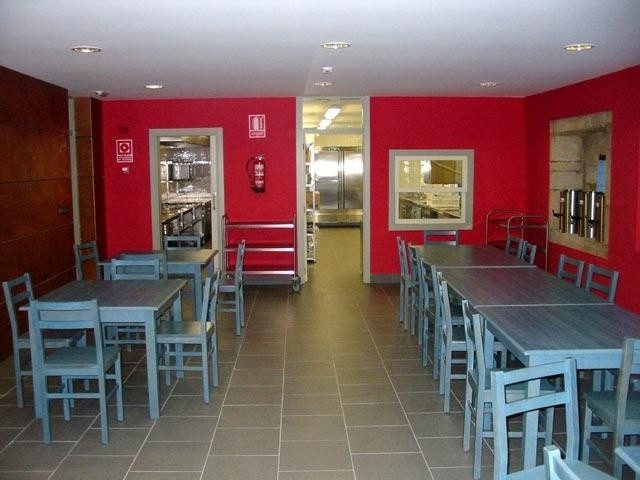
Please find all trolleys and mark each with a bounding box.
[485,207,548,273]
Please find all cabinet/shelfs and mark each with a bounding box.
[303,133,316,262]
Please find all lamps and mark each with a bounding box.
[318,105,342,130]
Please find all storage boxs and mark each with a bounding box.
[306,191,320,210]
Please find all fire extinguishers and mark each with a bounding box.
[246,152,266,193]
